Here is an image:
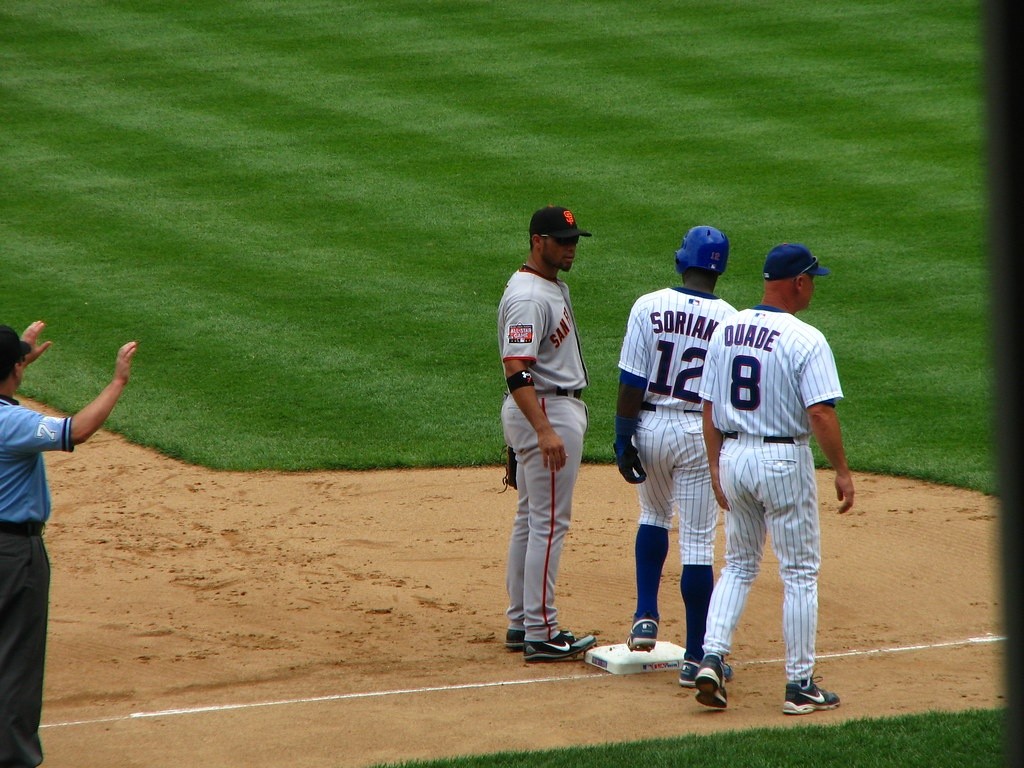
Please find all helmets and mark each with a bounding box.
[675,226,729,274]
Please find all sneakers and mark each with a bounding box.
[627,613,658,652]
[505,629,525,649]
[782,669,840,715]
[695,653,728,708]
[679,660,732,687]
[523,630,597,663]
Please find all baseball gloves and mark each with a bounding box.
[505,445,518,490]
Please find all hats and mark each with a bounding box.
[0,324,32,360]
[529,206,592,238]
[763,243,829,280]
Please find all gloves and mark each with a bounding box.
[614,434,647,484]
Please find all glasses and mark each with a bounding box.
[794,256,818,280]
[539,235,579,245]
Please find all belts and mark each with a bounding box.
[640,401,700,412]
[0,521,43,536]
[556,387,581,399]
[725,431,794,443]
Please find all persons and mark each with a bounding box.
[614,225,739,687]
[1,319,136,767]
[694,244,855,715]
[498,203,597,665]
